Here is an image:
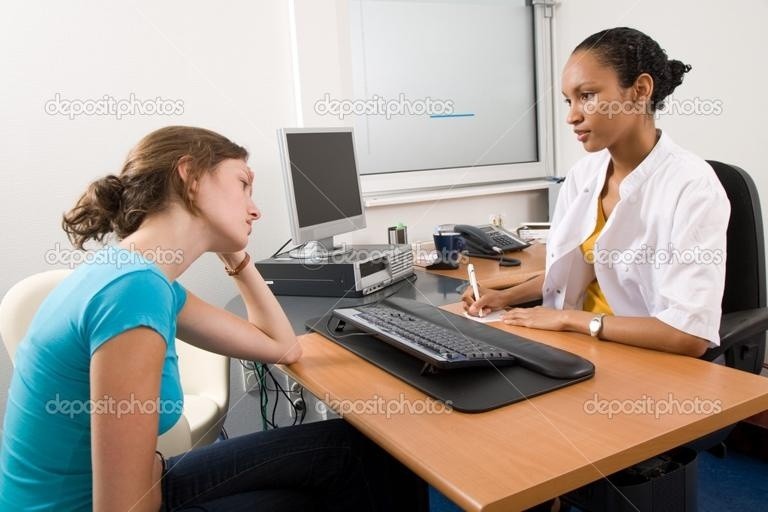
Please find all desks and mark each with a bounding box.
[222,230,768,511]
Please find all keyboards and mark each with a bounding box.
[333,303,513,377]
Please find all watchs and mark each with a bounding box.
[589,313,607,337]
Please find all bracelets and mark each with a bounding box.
[155,451,165,472]
[224,252,250,276]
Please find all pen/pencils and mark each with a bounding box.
[468,263,484,318]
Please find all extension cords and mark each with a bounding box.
[241,359,261,392]
[287,375,304,417]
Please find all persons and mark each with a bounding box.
[461,26,732,359]
[0,124,430,512]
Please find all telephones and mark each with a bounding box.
[454,224,532,256]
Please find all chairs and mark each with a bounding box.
[674,160,768,458]
[3,269,232,461]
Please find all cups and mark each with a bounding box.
[432,231,466,268]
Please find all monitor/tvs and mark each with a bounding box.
[276,126,367,258]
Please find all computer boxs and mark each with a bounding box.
[253,244,414,298]
